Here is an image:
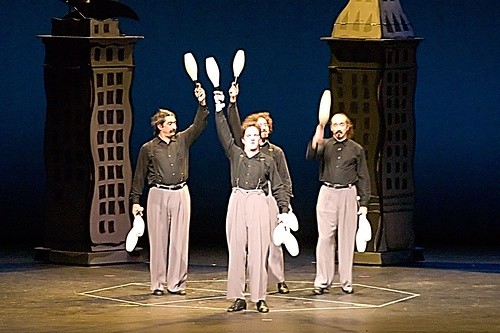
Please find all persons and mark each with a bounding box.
[305,111,372,294]
[212,82,293,313]
[131,83,210,296]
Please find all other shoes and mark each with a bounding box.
[227,298,247,312]
[277,281,289,294]
[176,290,186,295]
[256,300,269,313]
[342,286,354,294]
[311,288,330,295]
[153,290,164,296]
[244,282,252,294]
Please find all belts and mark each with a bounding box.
[153,183,187,190]
[322,182,354,189]
